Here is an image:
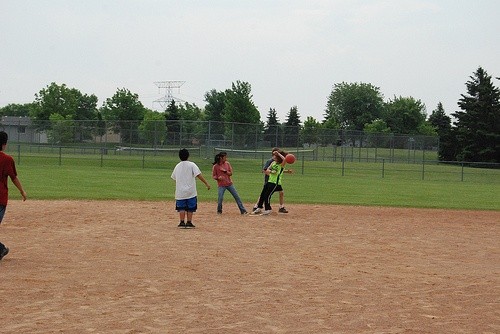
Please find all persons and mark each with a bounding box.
[252,147,292,214]
[1,130,26,261]
[212,152,250,215]
[170,148,211,229]
[251,150,288,215]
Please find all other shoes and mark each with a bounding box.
[0,244,9,260]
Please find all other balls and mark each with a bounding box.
[285,153,296,164]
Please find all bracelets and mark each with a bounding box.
[224,169,227,173]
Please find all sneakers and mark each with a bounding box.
[216,210,223,215]
[278,207,288,214]
[248,208,273,216]
[241,211,249,215]
[252,206,264,211]
[178,222,195,229]
